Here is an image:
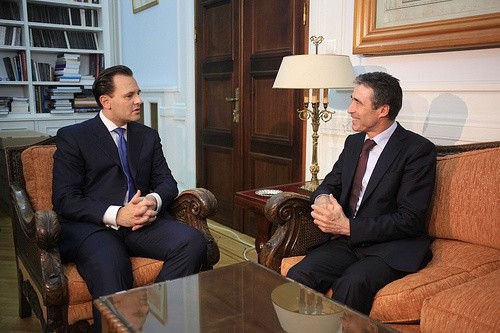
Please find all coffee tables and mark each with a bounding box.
[93,259,402,333]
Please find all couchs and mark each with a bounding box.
[3,135,221,333]
[257,140,500,333]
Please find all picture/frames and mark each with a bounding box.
[131,0,160,15]
[352,0,500,56]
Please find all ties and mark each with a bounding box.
[113,127,137,203]
[346,139,377,218]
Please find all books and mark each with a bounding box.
[0,0,105,114]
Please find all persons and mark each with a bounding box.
[340,309,377,333]
[108,287,153,332]
[52,65,207,333]
[285,72,437,317]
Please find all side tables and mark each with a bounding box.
[233,178,330,263]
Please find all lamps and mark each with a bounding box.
[271,280,347,333]
[272,36,358,192]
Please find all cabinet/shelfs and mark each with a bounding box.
[0,0,120,137]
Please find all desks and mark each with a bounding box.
[0,128,51,152]
